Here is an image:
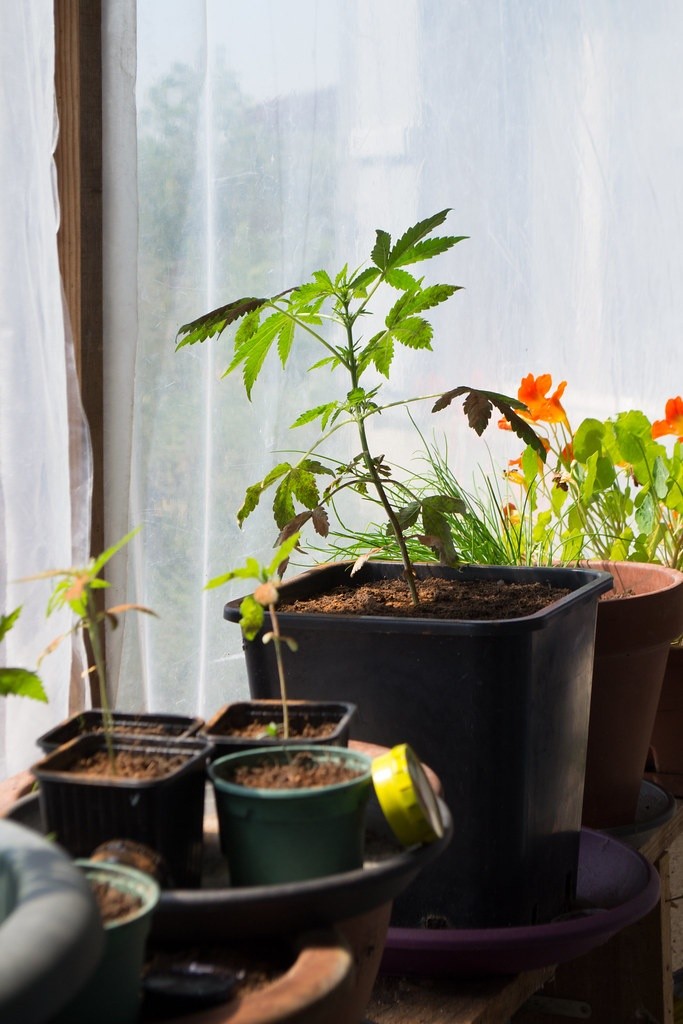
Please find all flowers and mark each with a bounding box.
[498,369,683,575]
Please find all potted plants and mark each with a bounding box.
[287,370,683,841]
[10,514,373,888]
[170,204,661,982]
